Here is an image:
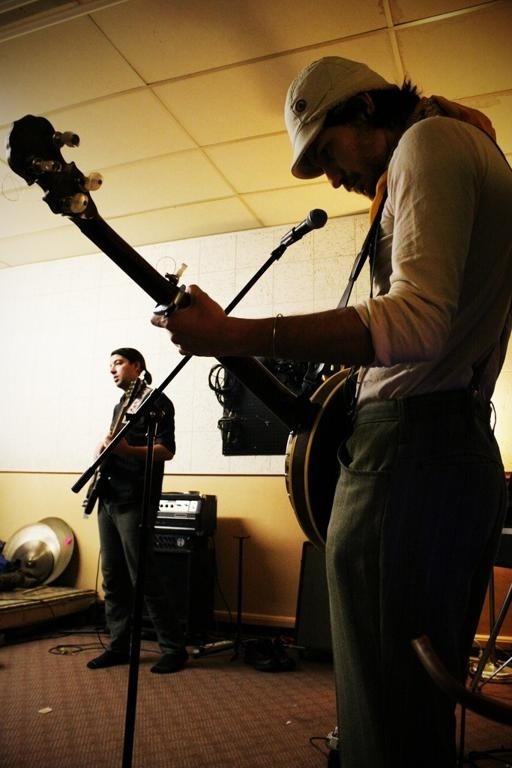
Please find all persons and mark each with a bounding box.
[87,347,192,675]
[150,55,510,767]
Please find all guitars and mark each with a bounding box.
[83,376,145,515]
[4,113,360,547]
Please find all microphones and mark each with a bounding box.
[281,209,328,245]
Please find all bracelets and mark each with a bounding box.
[268,311,289,365]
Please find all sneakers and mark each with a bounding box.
[150,653,188,674]
[86,649,130,670]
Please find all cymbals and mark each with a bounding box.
[3,516,76,593]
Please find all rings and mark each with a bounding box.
[159,313,169,329]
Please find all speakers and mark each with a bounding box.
[145,533,217,646]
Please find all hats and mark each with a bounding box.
[284,56,392,179]
